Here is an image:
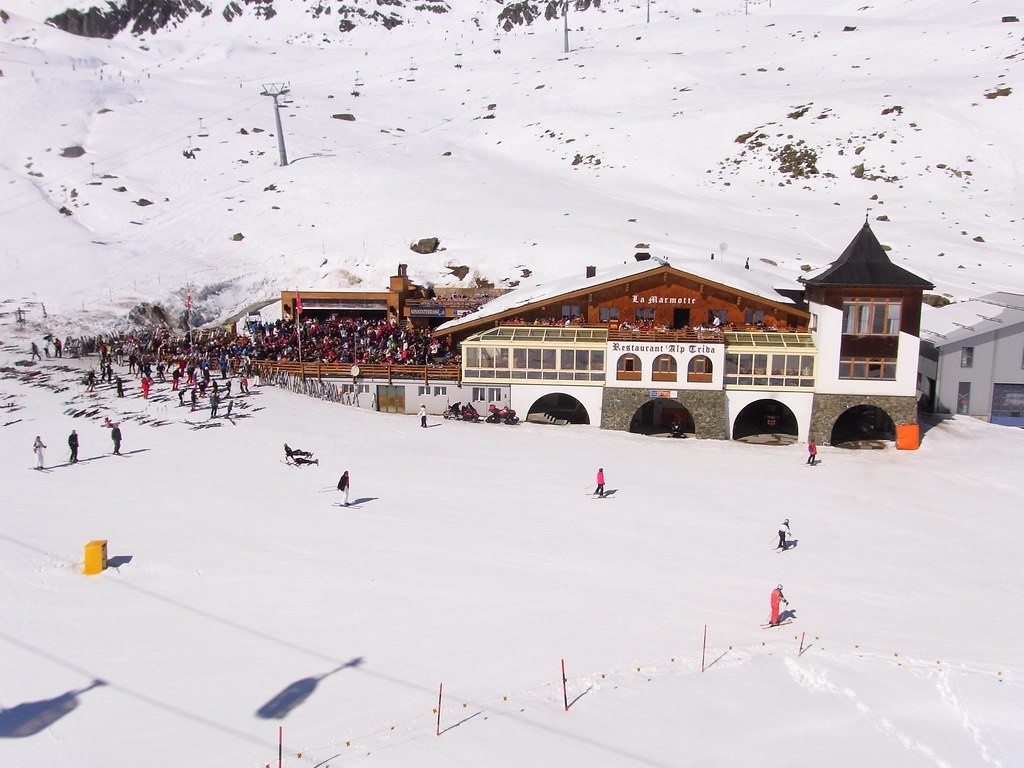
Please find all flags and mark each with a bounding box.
[187,289,193,311]
[296,289,303,313]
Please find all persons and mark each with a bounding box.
[111,423,123,456]
[105,417,118,428]
[594,468,605,498]
[465,402,474,411]
[33,436,46,469]
[778,519,791,551]
[808,439,817,466]
[770,584,789,626]
[416,291,499,306]
[416,403,428,428]
[283,443,297,465]
[68,430,79,463]
[337,471,352,507]
[499,315,793,335]
[30,313,462,419]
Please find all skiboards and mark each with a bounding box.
[330,500,364,510]
[280,460,301,468]
[32,466,54,474]
[772,545,791,555]
[106,451,133,459]
[802,463,826,468]
[761,621,793,629]
[585,493,616,499]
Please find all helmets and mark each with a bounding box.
[785,519,789,522]
[778,584,783,589]
